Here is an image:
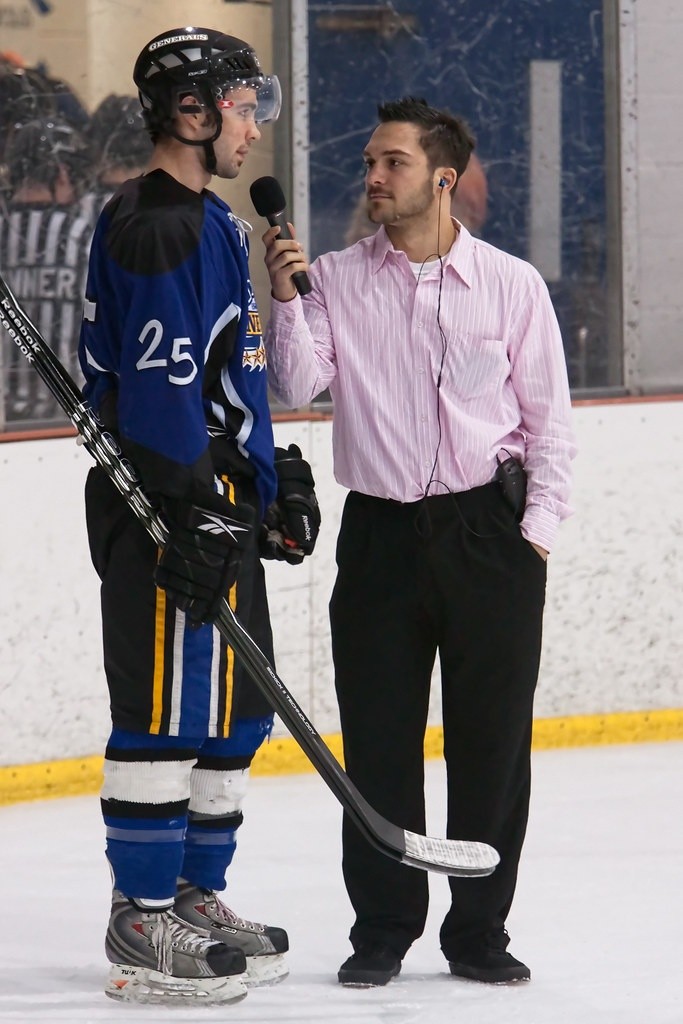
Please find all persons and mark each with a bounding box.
[77,26,290,1007]
[0,89,158,422]
[261,94,577,986]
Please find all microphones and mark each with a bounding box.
[250,176,312,296]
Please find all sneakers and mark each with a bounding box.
[338,952,402,987]
[449,952,531,984]
[105,889,248,1009]
[175,876,290,989]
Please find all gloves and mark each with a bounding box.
[154,490,257,624]
[259,444,321,565]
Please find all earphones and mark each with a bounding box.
[439,179,446,187]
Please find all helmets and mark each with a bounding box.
[133,27,282,124]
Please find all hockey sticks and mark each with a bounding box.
[0,266,502,878]
